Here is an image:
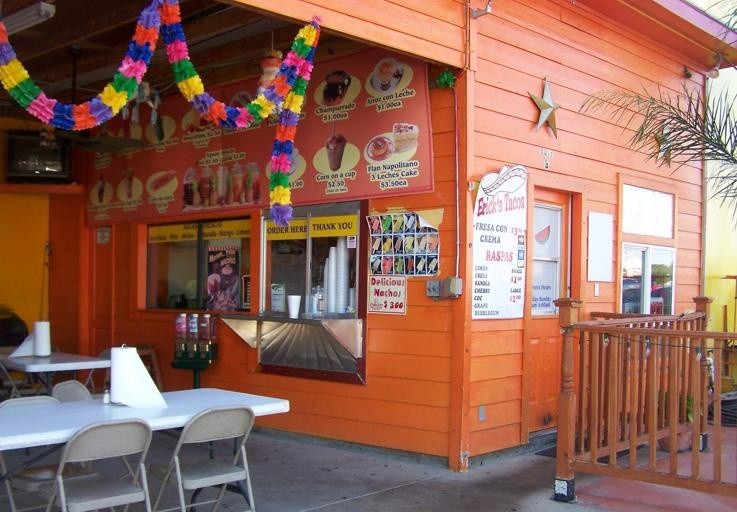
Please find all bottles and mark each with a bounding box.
[102,389,110,404]
[310,285,324,314]
[184,162,261,207]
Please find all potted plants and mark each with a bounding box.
[652,389,704,453]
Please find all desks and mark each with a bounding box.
[0,385,289,510]
[0,351,110,395]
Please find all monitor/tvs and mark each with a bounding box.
[6,129,72,184]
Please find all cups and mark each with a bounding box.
[324,237,349,313]
[287,295,302,319]
[349,288,355,310]
[326,137,347,171]
[323,70,352,106]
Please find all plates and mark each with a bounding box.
[313,141,360,174]
[90,171,179,207]
[364,63,414,100]
[93,115,176,157]
[313,75,362,108]
[265,153,307,183]
[181,109,215,134]
[363,132,418,168]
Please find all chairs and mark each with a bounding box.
[0,391,261,512]
